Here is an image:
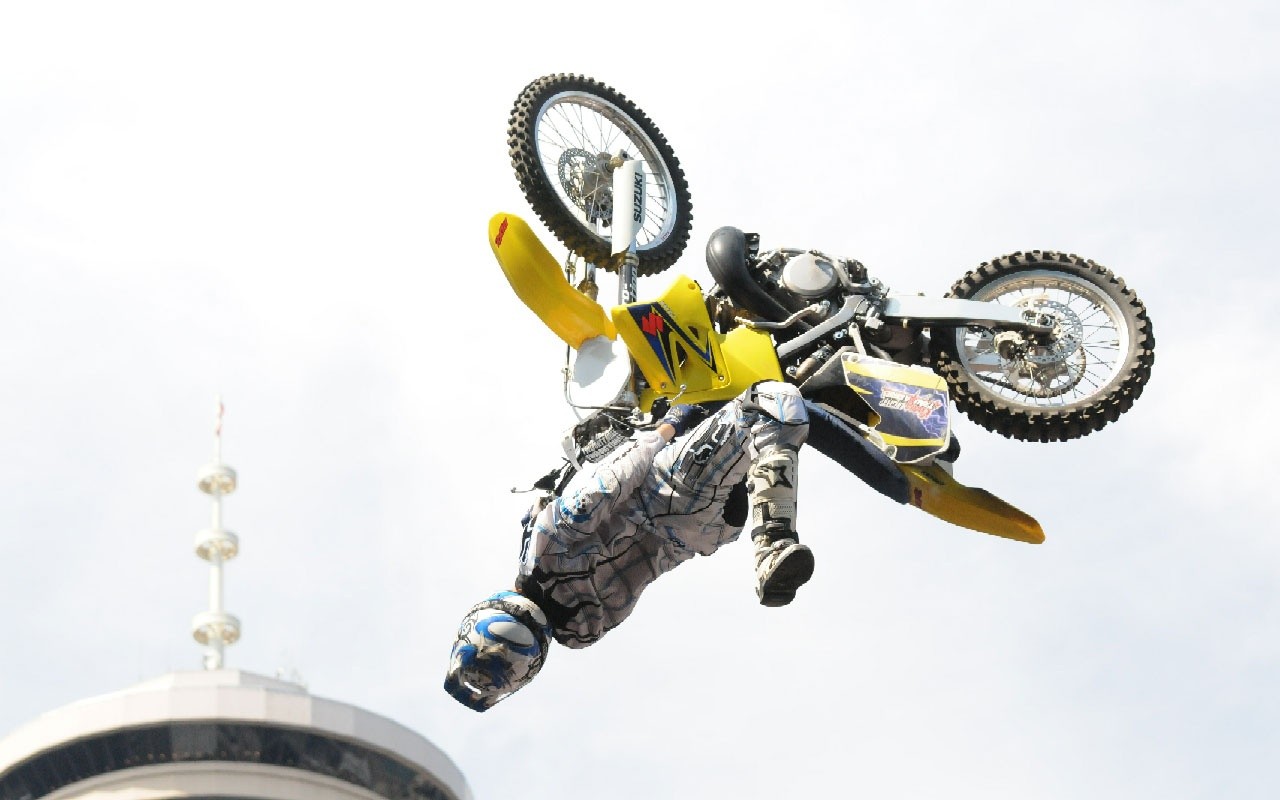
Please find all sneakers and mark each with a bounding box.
[754,539,815,607]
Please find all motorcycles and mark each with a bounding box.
[487,73,1157,545]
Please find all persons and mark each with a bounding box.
[443,380,823,713]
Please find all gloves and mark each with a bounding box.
[663,403,704,437]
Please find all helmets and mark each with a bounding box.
[442,593,552,711]
